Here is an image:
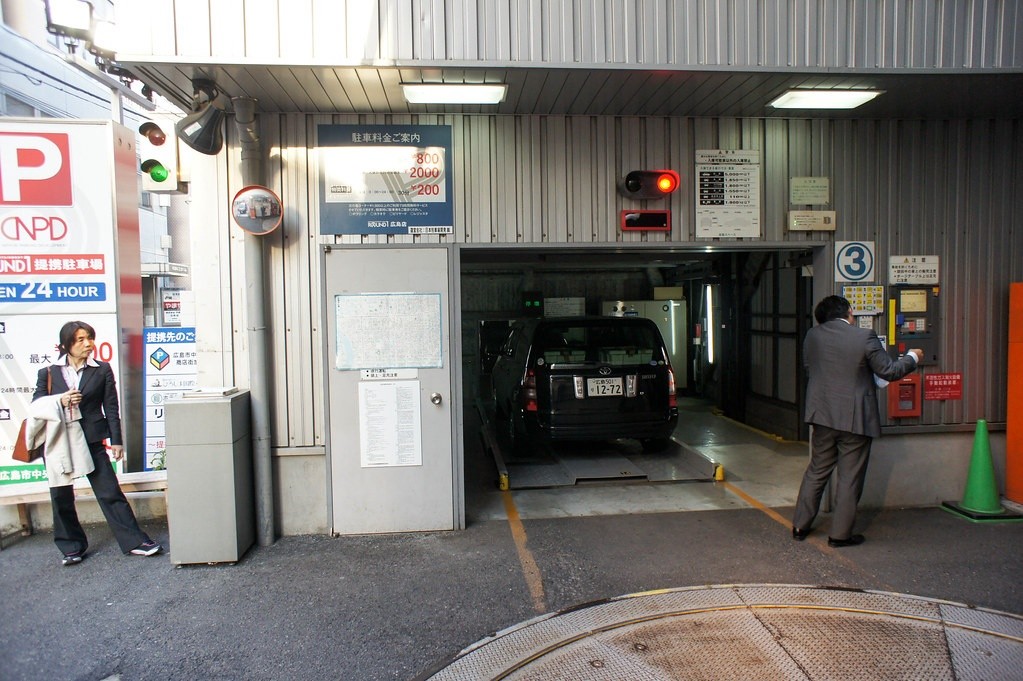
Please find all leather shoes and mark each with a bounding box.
[828,535,865,547]
[794,527,813,539]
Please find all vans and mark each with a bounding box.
[490,313,679,457]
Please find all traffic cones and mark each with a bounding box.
[938,419,1023,524]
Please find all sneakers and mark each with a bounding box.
[130,540,164,557]
[61,554,81,565]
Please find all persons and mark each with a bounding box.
[792,294,924,549]
[31,319,163,564]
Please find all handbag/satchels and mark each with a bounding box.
[12,366,51,463]
